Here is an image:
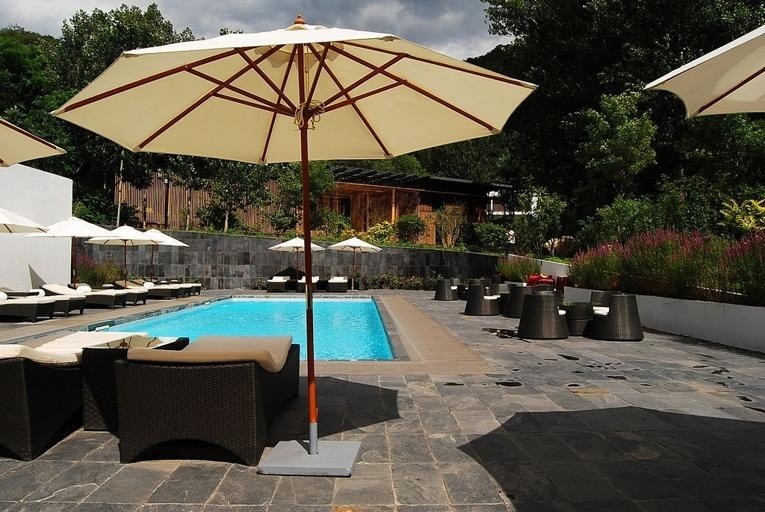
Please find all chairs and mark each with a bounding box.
[505,286,532,316]
[67,283,149,306]
[39,284,127,310]
[0,331,148,460]
[113,280,181,299]
[131,280,202,296]
[328,276,348,293]
[298,276,319,293]
[517,294,568,338]
[30,290,86,317]
[590,290,620,310]
[114,336,300,467]
[267,276,290,292]
[594,295,643,341]
[435,279,457,301]
[464,285,500,315]
[1,293,57,323]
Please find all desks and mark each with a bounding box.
[79,336,189,431]
[561,302,594,336]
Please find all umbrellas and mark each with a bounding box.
[0,209,49,234]
[327,236,383,290]
[49,13,539,455]
[145,228,189,282]
[0,117,67,168]
[268,235,326,280]
[24,216,119,289]
[644,25,765,124]
[83,226,165,289]
